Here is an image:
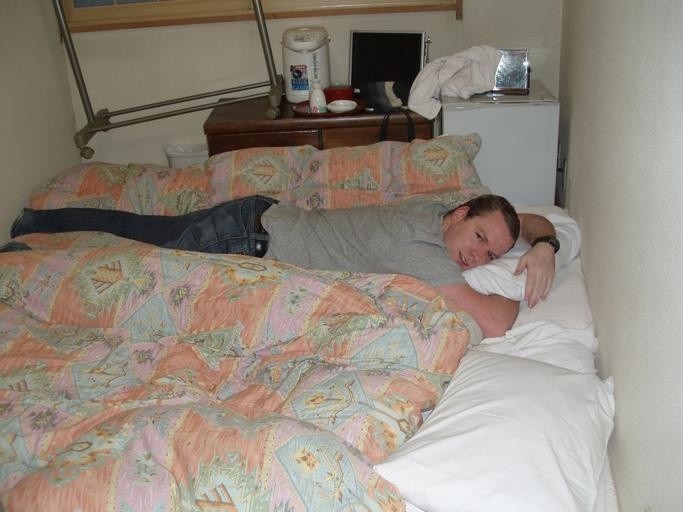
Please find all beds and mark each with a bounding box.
[0,133,620,512]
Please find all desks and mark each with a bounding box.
[204,96,435,158]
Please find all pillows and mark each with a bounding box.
[373,346,615,512]
[462,213,582,301]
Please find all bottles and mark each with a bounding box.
[310,84,327,113]
[325,85,354,103]
[308,78,320,101]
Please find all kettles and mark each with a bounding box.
[281,25,331,103]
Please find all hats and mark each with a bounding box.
[355,80,406,113]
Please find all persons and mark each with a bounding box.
[0,194,556,338]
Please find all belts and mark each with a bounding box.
[254,200,266,258]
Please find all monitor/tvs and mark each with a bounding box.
[349,32,425,112]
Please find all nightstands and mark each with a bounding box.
[440,78,560,206]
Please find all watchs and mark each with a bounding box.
[532,235,560,254]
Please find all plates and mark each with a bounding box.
[328,99,357,113]
